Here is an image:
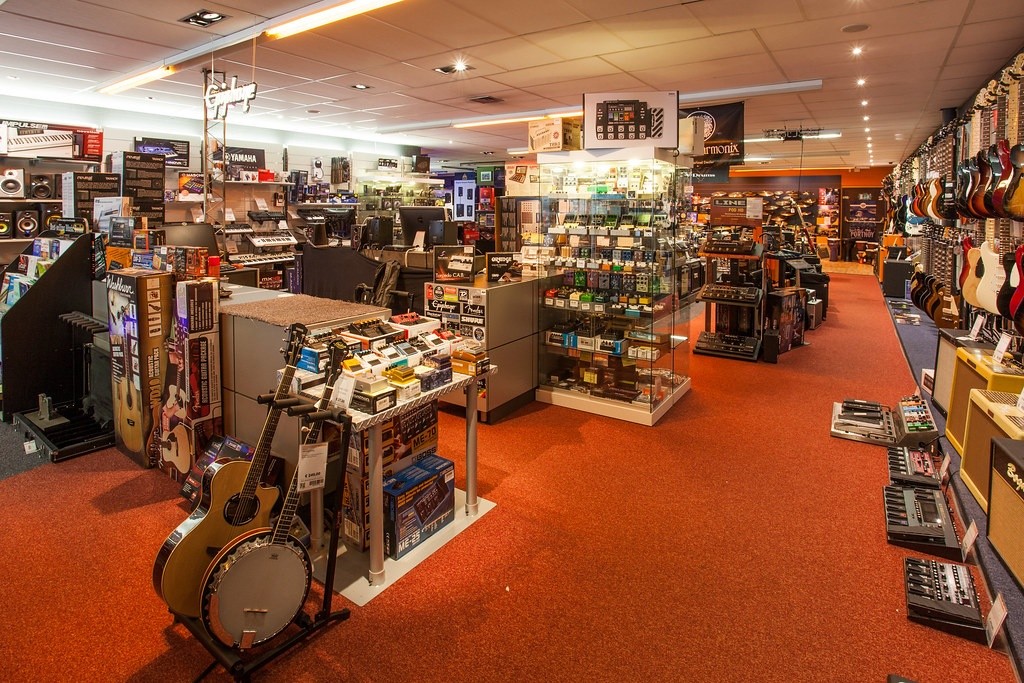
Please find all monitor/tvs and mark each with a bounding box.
[324,209,356,239]
[399,206,448,251]
[165,222,220,259]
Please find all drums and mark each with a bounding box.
[842,237,856,262]
[855,240,868,264]
[826,238,841,261]
[867,242,878,266]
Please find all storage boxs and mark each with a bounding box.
[770,286,812,352]
[543,199,678,407]
[298,311,494,558]
[0,119,291,506]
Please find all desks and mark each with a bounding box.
[303,365,499,609]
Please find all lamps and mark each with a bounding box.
[743,130,841,142]
[266,0,402,42]
[744,154,774,163]
[101,66,180,94]
[453,108,585,129]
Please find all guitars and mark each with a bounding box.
[118,305,144,453]
[890,52,1024,223]
[198,334,357,651]
[151,321,309,620]
[155,423,192,478]
[166,356,188,419]
[909,217,1024,338]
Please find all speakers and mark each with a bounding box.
[352,216,393,249]
[0,169,63,240]
[931,327,1024,589]
[428,220,457,247]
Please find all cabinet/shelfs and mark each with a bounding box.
[422,277,537,421]
[0,231,95,421]
[0,160,101,243]
[693,198,765,360]
[534,156,693,427]
